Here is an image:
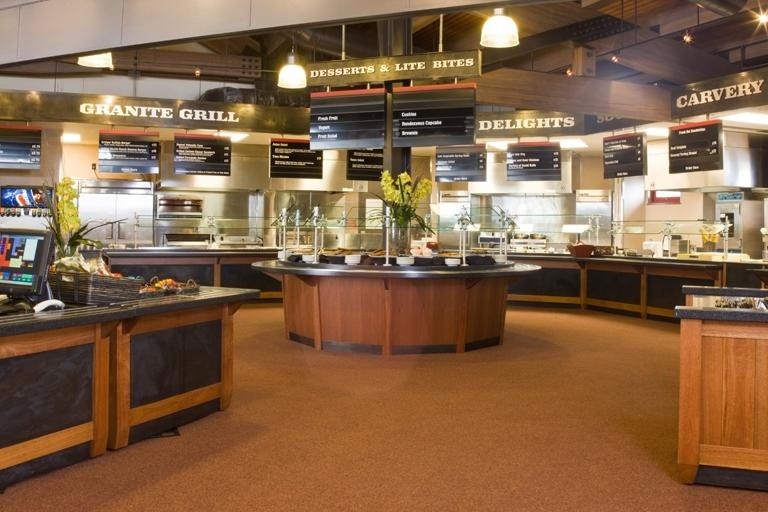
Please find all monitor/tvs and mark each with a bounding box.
[0,228,55,296]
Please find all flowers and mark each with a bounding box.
[20,168,128,281]
[345,167,436,236]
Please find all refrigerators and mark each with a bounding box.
[78,181,153,248]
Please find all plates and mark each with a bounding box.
[278,249,461,266]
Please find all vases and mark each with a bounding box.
[50,245,90,284]
[389,218,411,256]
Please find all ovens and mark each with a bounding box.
[714,199,763,259]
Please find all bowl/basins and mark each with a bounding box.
[565,243,614,258]
[514,233,546,239]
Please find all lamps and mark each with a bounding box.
[277,29,307,89]
[78,51,113,69]
[480,7,520,49]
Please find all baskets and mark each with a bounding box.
[46,268,144,306]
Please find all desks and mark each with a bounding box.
[251,249,543,357]
